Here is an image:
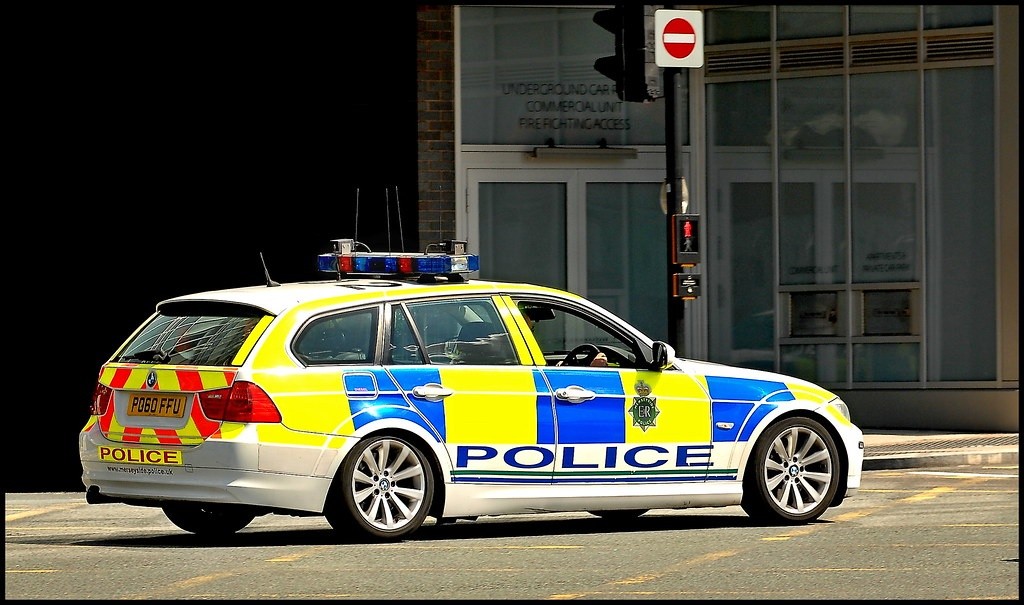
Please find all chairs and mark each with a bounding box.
[450,322,511,364]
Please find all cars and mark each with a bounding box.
[77,235,866,543]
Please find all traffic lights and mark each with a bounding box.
[592,0,649,102]
[672,212,700,266]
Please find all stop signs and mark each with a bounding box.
[654,8,705,69]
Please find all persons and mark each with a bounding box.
[494,313,608,367]
